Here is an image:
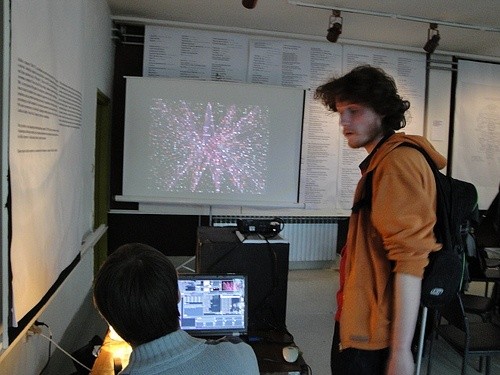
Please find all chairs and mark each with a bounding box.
[414,209,500,375]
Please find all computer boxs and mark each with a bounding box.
[196,226,289,332]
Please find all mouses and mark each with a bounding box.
[282,346,299,363]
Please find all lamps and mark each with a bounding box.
[424,22,442,53]
[326,9,344,43]
[241,0,259,9]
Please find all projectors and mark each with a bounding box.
[238,218,281,233]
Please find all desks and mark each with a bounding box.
[91,315,309,375]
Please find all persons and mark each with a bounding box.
[312,66,448,375]
[94,243,262,375]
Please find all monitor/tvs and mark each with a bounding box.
[174,273,249,342]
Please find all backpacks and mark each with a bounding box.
[352,142,478,310]
